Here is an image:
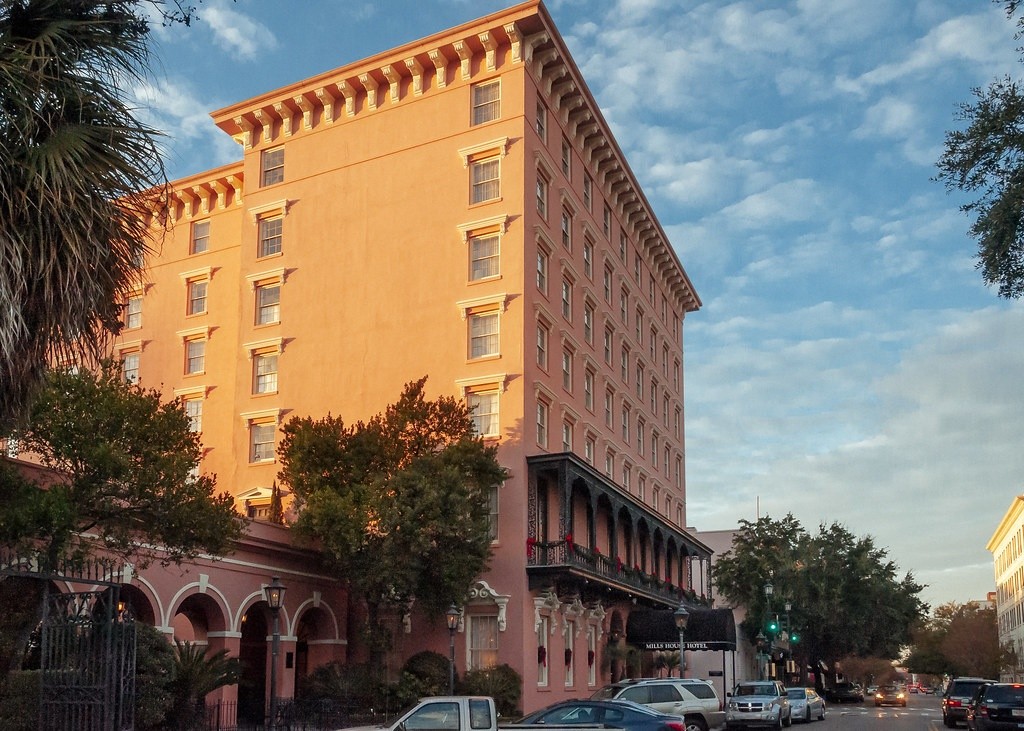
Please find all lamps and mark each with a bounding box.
[240,611,248,626]
[113,593,128,617]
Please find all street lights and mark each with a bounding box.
[444,601,461,696]
[783,599,793,687]
[262,575,289,731]
[673,600,690,679]
[763,579,775,680]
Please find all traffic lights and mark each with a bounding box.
[792,632,797,643]
[770,620,776,633]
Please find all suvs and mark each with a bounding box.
[961,681,1024,731]
[560,677,727,731]
[937,677,999,729]
[725,680,792,731]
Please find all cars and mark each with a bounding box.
[909,687,918,694]
[509,696,686,731]
[829,681,860,704]
[784,687,826,724]
[873,685,907,707]
[867,684,880,696]
[854,683,865,702]
[915,684,935,695]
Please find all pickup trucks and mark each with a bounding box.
[336,695,625,731]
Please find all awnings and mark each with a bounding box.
[625,609,734,650]
